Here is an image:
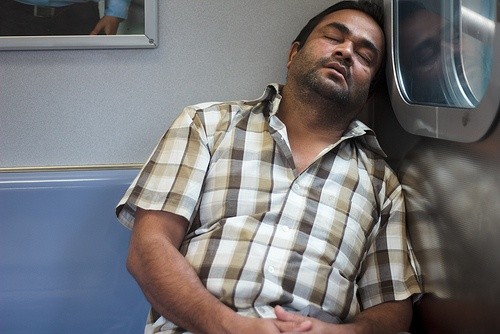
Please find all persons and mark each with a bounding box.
[397,0,483,106]
[401,142,500,334]
[115,0,425,334]
[1,0,134,36]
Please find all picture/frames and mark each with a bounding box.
[0,0,159,49]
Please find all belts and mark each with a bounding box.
[6,0,98,18]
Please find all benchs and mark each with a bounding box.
[0,167,150,334]
